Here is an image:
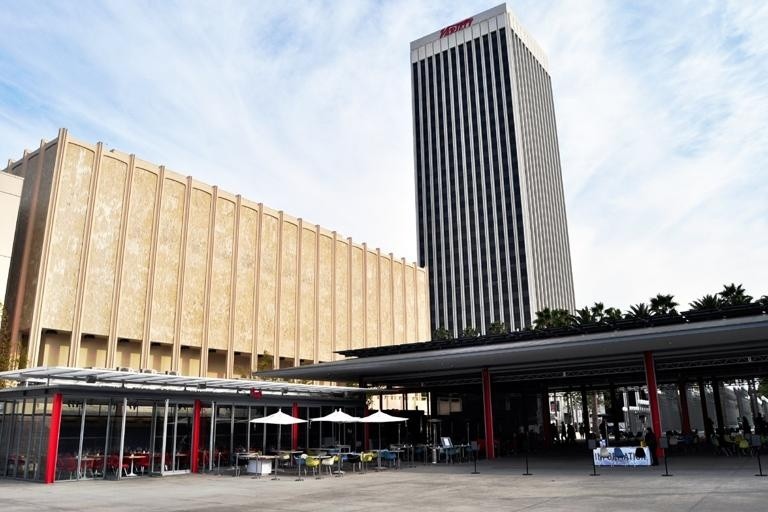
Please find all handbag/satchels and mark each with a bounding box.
[752,435,761,446]
[735,435,749,448]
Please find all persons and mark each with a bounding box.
[578,424,585,438]
[308,407,366,471]
[359,407,411,467]
[644,427,660,466]
[560,421,568,440]
[566,423,575,441]
[551,423,560,441]
[703,411,768,460]
[248,407,309,454]
[599,420,608,434]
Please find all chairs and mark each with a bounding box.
[711,434,767,457]
